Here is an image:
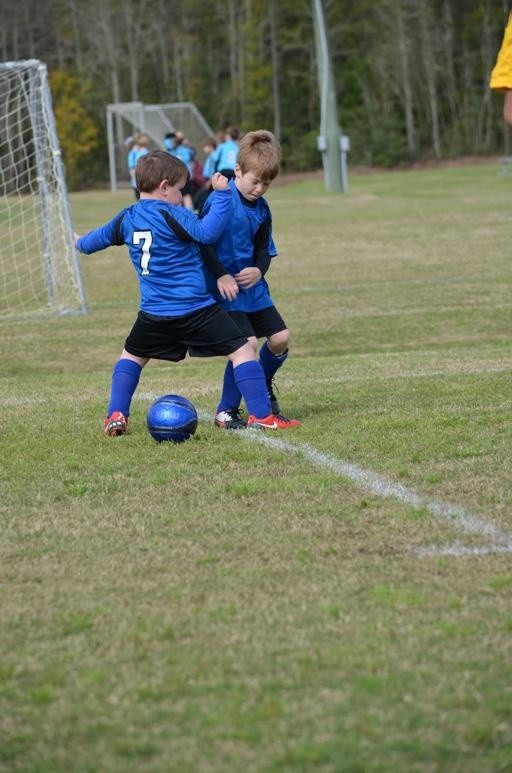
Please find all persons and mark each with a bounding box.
[125,125,245,210]
[73,151,301,437]
[199,128,291,431]
[488,12,511,126]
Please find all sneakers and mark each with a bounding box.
[266,374,282,414]
[215,407,248,429]
[247,412,302,430]
[104,412,128,436]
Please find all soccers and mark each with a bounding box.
[147,394,198,442]
